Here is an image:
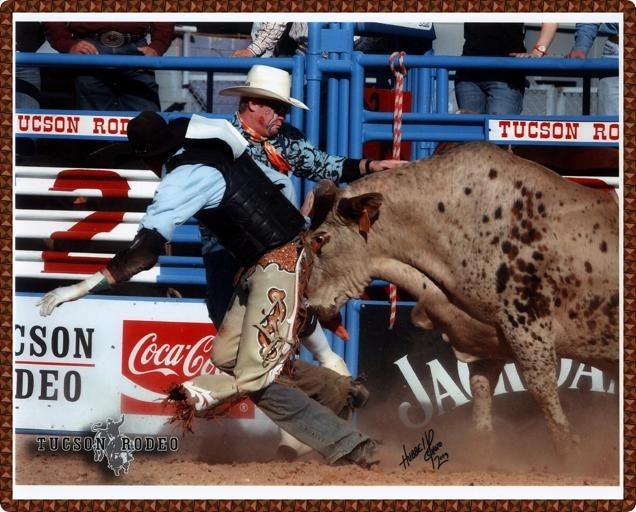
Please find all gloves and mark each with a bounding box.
[35,285,79,316]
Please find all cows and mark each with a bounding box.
[299,138,619,475]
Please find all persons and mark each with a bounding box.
[33,108,380,471]
[563,22,619,116]
[42,23,175,169]
[453,23,559,115]
[231,23,309,57]
[198,65,415,464]
[15,22,51,166]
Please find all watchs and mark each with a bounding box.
[532,44,548,56]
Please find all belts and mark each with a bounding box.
[608,33,619,44]
[73,31,149,48]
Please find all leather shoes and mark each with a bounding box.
[340,383,369,418]
[345,441,384,469]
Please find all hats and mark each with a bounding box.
[219,64,309,111]
[127,111,169,142]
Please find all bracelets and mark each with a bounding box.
[364,158,374,174]
